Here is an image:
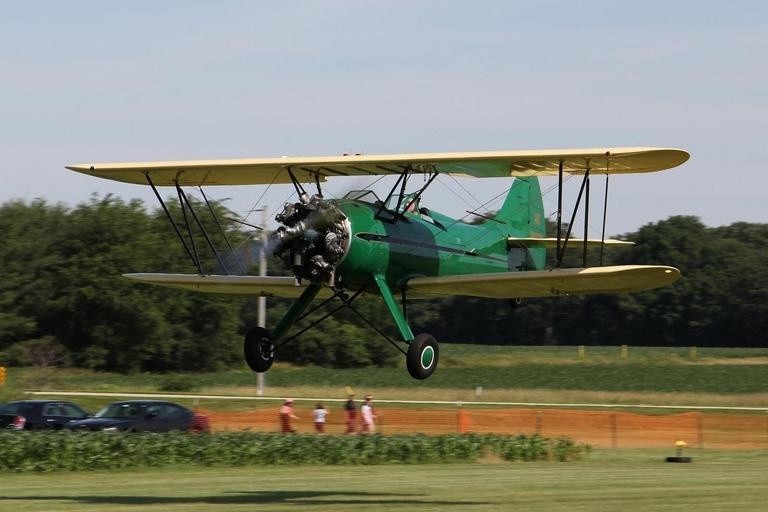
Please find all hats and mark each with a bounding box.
[285,398,294,403]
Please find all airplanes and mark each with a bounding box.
[64,147,690,380]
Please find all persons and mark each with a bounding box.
[404,194,435,224]
[279,393,379,436]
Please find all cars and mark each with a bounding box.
[0,400,211,432]
